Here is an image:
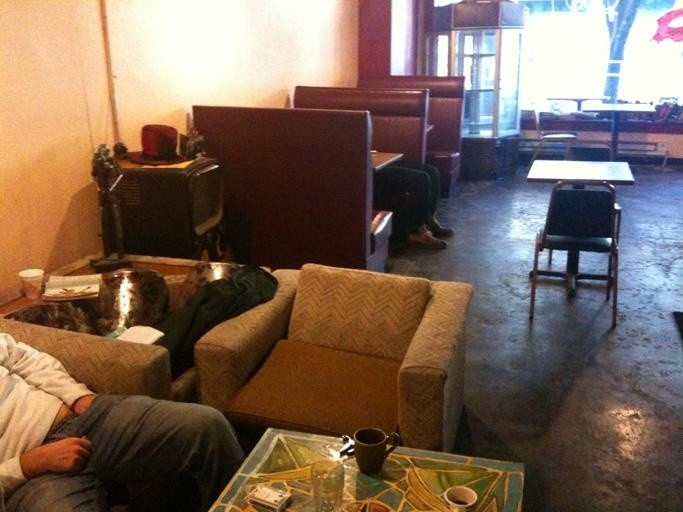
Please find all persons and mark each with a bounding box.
[0,334,246,512]
[383,160,455,249]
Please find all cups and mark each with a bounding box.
[310,458,347,512]
[442,486,478,512]
[353,427,400,476]
[17,268,43,300]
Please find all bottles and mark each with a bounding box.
[185,128,206,157]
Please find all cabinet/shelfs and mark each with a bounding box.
[428,0,526,181]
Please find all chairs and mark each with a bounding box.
[527,104,577,167]
[0,316,175,406]
[528,180,622,331]
[194,262,474,456]
[546,143,621,270]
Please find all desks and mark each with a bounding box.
[0,246,274,403]
[545,97,611,112]
[580,102,657,161]
[522,158,637,299]
[207,426,530,512]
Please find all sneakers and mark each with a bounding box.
[409,234,447,249]
[430,222,454,239]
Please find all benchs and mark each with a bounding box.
[192,105,393,273]
[294,75,466,229]
